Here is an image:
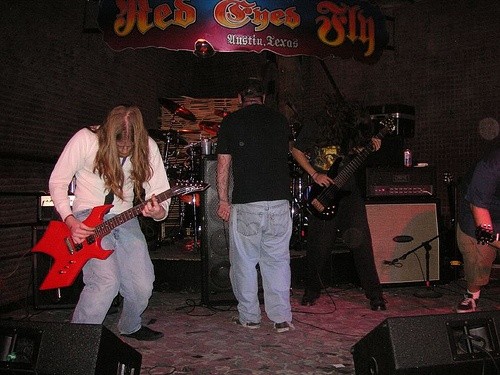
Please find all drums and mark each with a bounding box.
[161,154,206,208]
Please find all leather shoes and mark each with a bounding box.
[370,295,387,311]
[302,292,319,305]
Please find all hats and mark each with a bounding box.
[240,78,263,97]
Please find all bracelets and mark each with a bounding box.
[311,172,317,177]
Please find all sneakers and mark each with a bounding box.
[456,290,480,313]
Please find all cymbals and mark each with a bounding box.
[147,129,188,144]
[160,97,197,121]
[213,107,230,117]
[200,120,222,133]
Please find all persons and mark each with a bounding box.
[456,135,500,313]
[216,71,335,334]
[48,104,172,342]
[292,101,387,311]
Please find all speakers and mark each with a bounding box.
[197,155,263,306]
[364,195,444,286]
[30,229,116,310]
[349,311,500,375]
[0,318,143,375]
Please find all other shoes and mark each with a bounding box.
[120,325,164,341]
[275,322,289,333]
[232,318,261,328]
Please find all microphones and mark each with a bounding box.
[382,259,393,266]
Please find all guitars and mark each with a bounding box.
[301,113,399,221]
[31,177,212,291]
